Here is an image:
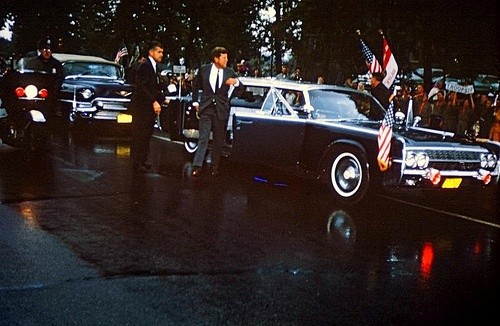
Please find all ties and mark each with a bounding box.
[215,70,219,92]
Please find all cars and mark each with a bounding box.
[0,53,135,142]
[156,76,499,208]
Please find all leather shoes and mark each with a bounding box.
[192,165,202,177]
[212,165,221,174]
[132,159,151,170]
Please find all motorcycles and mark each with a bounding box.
[3,68,57,155]
